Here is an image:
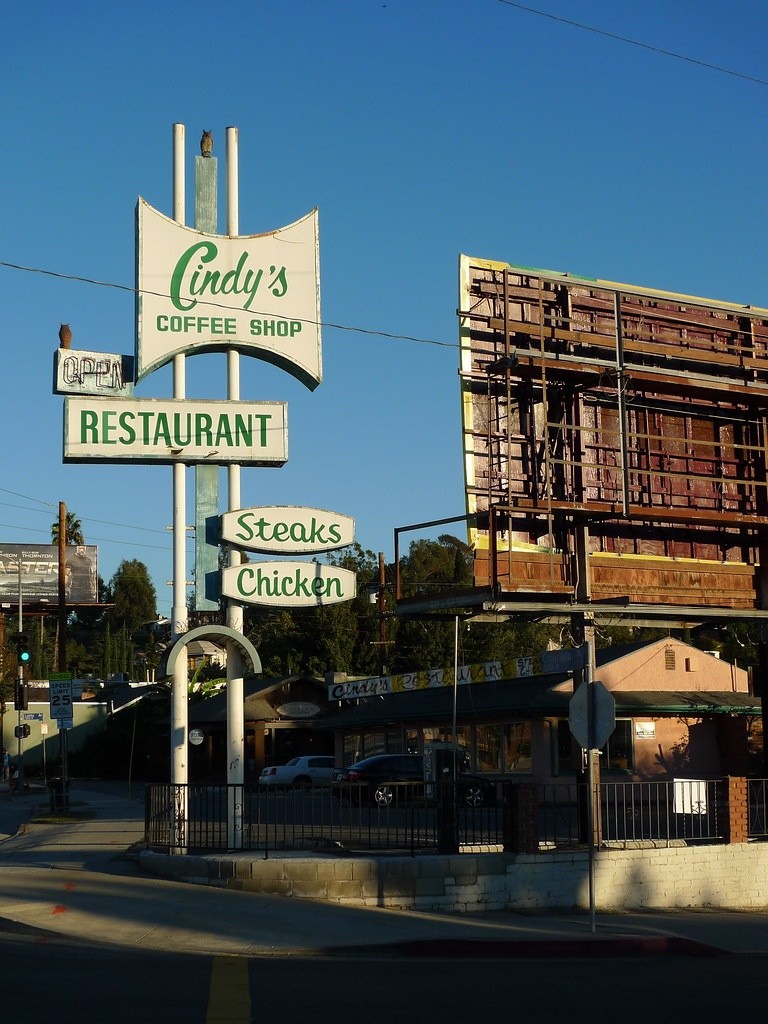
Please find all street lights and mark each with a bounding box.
[369,592,387,677]
[0,554,24,794]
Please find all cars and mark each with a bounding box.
[489,737,532,772]
[258,755,335,793]
[331,753,497,808]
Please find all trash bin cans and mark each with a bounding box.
[46,777,69,811]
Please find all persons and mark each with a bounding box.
[2,747,12,784]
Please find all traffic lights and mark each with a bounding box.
[15,726,22,737]
[17,632,30,666]
[23,724,30,737]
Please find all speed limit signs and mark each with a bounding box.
[50,680,73,719]
